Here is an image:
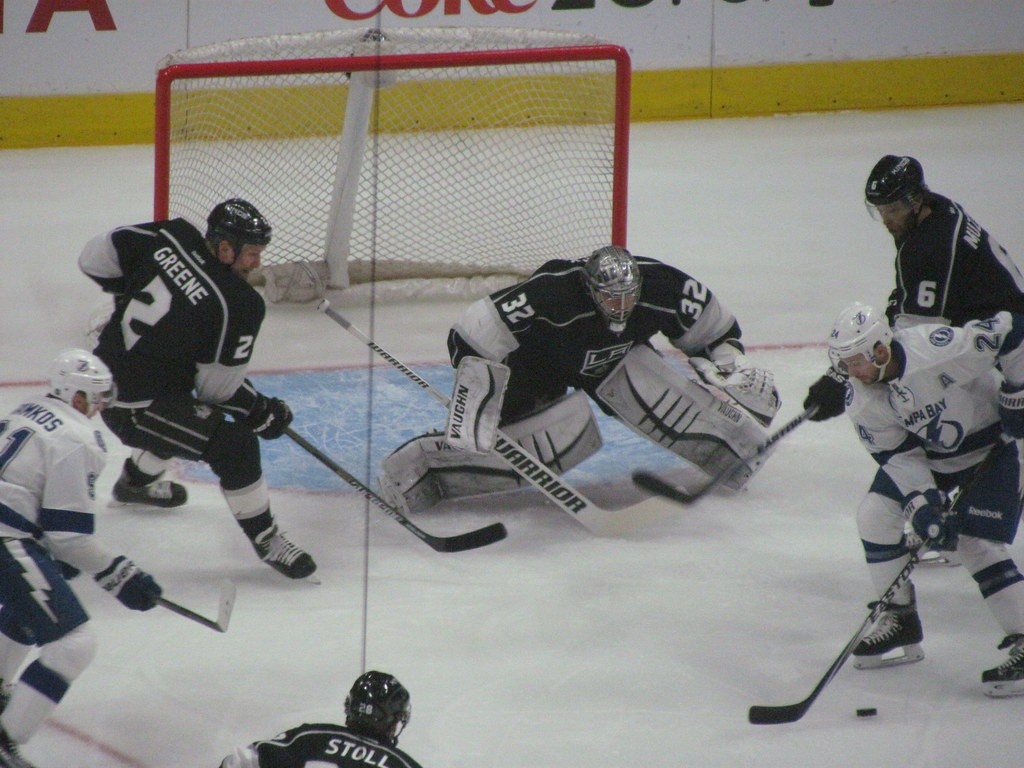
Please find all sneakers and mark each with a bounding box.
[244,517,322,585]
[108,458,187,509]
[982,633,1024,697]
[0,678,33,768]
[852,584,925,669]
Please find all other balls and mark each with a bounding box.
[857,708,877,717]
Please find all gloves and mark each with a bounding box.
[804,367,854,421]
[117,572,162,612]
[912,502,959,552]
[1000,381,1024,440]
[251,397,293,441]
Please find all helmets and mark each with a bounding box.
[50,350,113,419]
[827,303,892,368]
[865,154,924,205]
[344,670,410,735]
[580,245,644,325]
[208,198,273,245]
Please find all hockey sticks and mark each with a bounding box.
[632,401,822,505]
[144,580,236,633]
[317,299,693,536]
[284,425,508,553]
[749,478,974,726]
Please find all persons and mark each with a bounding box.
[384,246,780,521]
[804,154,1024,566]
[831,302,1024,698]
[218,672,422,768]
[78,199,323,586]
[1,348,164,768]
